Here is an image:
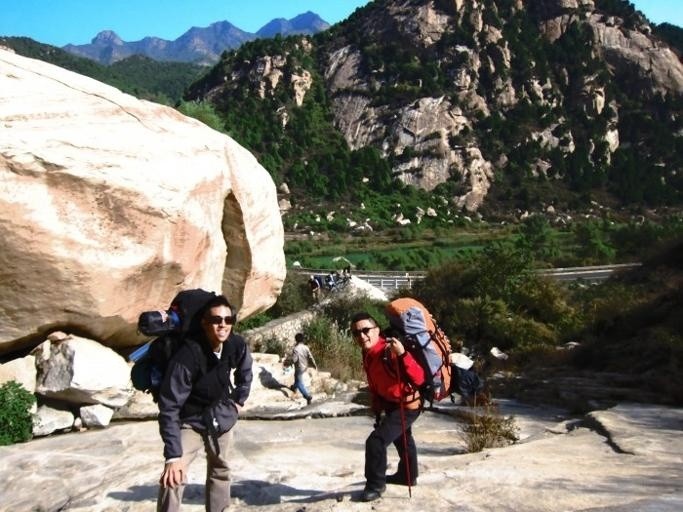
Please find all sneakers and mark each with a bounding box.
[385,472,417,485]
[362,484,386,501]
[307,396,312,404]
[291,385,296,392]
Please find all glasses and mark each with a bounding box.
[352,328,375,337]
[212,315,235,325]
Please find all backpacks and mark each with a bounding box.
[384,298,453,401]
[131,289,216,403]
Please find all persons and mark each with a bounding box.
[305,275,320,302]
[155,293,254,512]
[324,268,351,293]
[279,333,319,406]
[348,310,426,502]
